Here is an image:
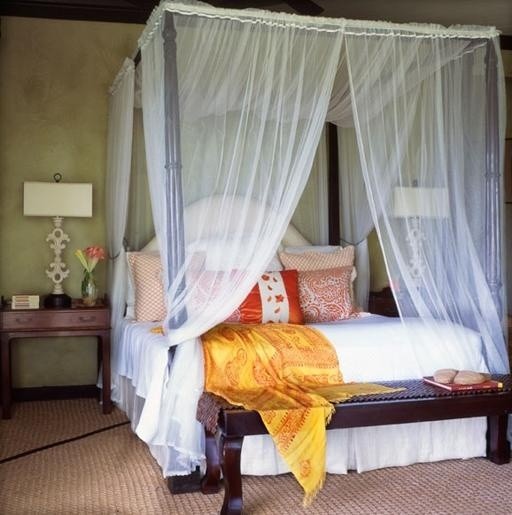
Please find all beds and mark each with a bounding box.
[97,194,491,494]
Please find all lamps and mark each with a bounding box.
[25,172,93,310]
[391,179,451,297]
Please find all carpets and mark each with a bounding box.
[2,398,510,514]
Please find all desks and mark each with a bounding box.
[1,297,111,421]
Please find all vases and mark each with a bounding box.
[81,272,97,307]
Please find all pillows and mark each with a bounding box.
[123,242,361,327]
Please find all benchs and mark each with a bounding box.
[196,372,511,515]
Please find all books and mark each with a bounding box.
[13,294,40,310]
[422,371,503,394]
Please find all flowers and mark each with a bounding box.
[73,246,106,272]
